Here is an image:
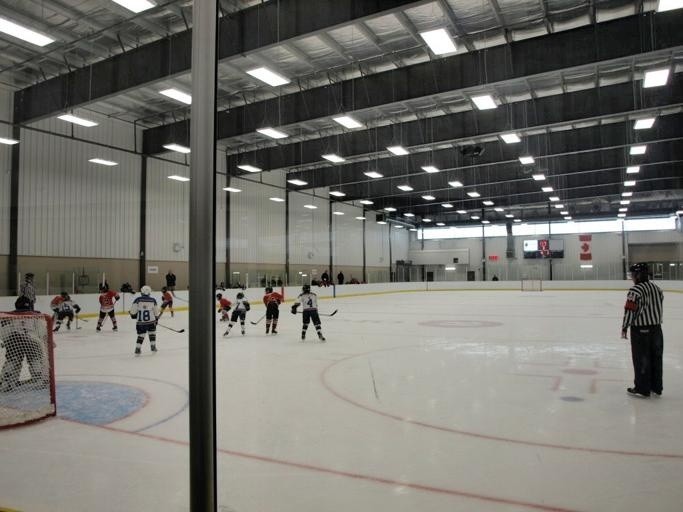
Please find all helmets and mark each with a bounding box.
[630,262,648,272]
[265,287,273,293]
[15,296,31,310]
[237,292,244,299]
[141,285,151,296]
[303,285,310,292]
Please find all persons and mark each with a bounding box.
[261,275,283,287]
[120,283,136,294]
[166,269,176,291]
[0,295,56,393]
[619,260,666,399]
[96,279,121,333]
[216,293,232,321]
[160,288,174,317]
[224,292,250,335]
[291,285,325,340]
[311,269,345,288]
[263,286,283,335]
[215,279,246,290]
[49,291,80,331]
[20,273,36,311]
[127,284,161,355]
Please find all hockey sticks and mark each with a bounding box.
[250,314,266,324]
[76,313,81,329]
[296,309,337,316]
[158,324,185,333]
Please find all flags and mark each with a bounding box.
[578,234,592,261]
